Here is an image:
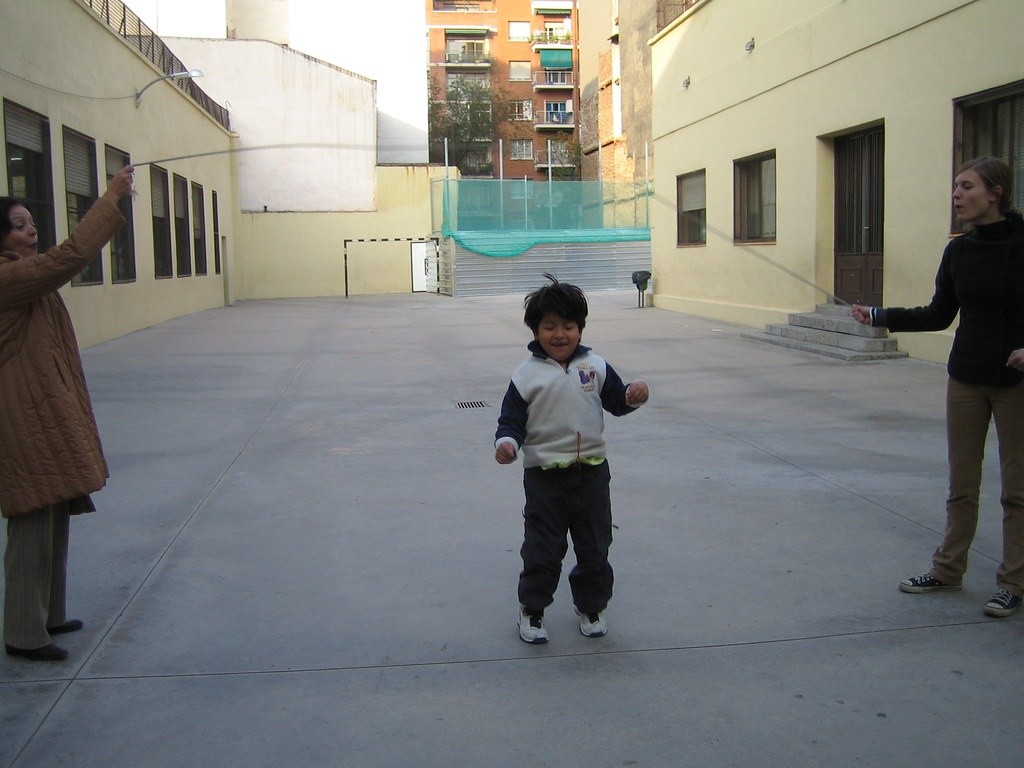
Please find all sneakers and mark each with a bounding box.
[47,619,84,635]
[516,606,549,642]
[984,588,1024,616]
[6,643,69,659]
[898,573,963,593]
[574,606,608,637]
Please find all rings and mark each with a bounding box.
[131,173,134,177]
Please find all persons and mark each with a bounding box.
[851,156,1024,618]
[494,272,649,644]
[0,165,135,661]
